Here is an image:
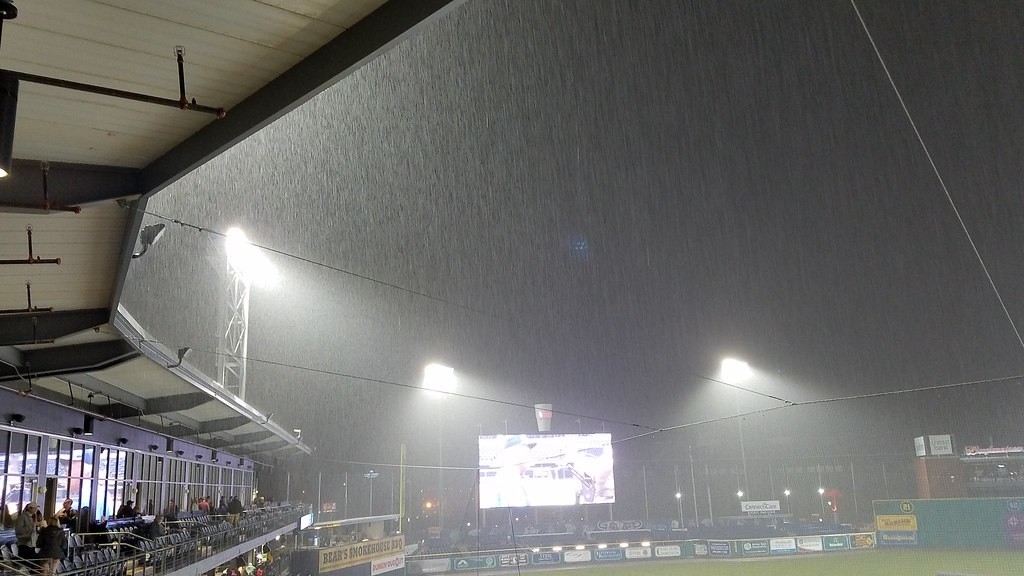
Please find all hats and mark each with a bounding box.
[127,500,134,504]
[28,502,40,509]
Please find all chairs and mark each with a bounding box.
[0,506,312,576]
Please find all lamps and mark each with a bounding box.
[10,224,317,470]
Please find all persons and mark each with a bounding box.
[0,495,312,576]
[494,434,541,508]
